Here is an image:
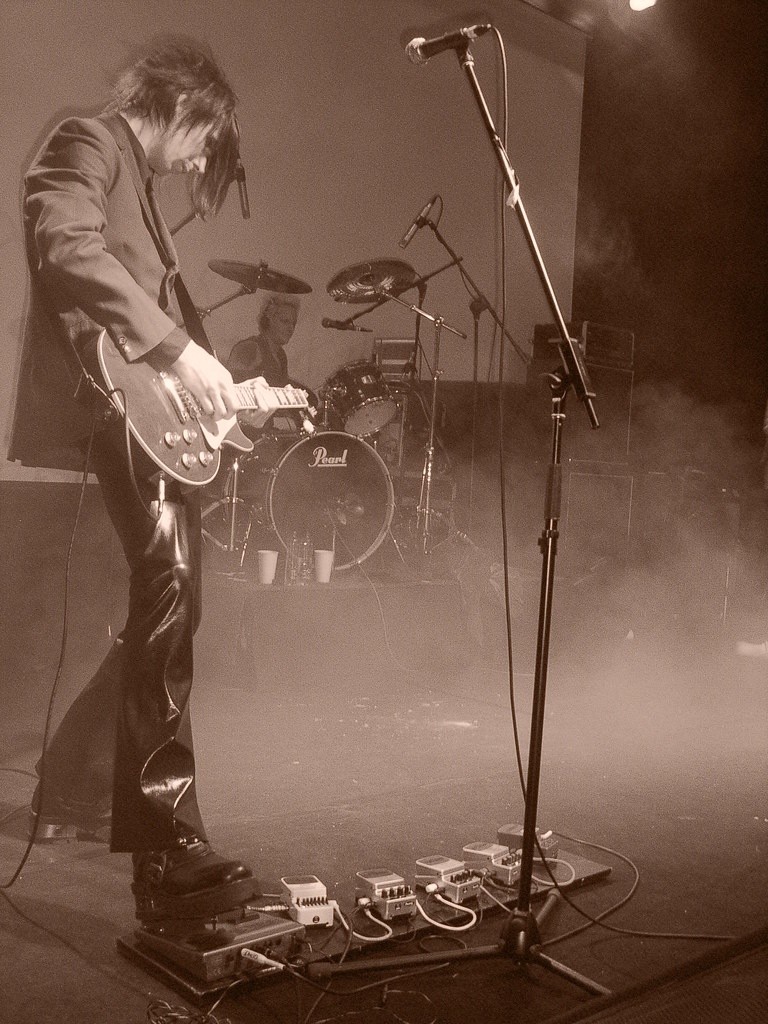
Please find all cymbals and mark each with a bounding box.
[324,257,416,305]
[207,257,313,295]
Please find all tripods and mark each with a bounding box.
[306,52,615,1000]
[202,455,274,576]
[365,278,487,586]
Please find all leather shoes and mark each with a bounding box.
[26,780,113,846]
[131,840,264,922]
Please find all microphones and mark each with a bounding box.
[398,194,438,249]
[322,318,373,333]
[404,23,493,66]
[234,155,252,220]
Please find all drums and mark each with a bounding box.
[321,358,399,438]
[222,429,397,573]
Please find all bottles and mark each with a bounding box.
[287,520,312,585]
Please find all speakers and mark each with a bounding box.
[526,359,738,635]
[540,925,768,1024]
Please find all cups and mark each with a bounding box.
[257,550,279,584]
[314,550,334,583]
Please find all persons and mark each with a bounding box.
[22,42,278,921]
[227,296,300,385]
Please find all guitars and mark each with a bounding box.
[80,325,312,504]
